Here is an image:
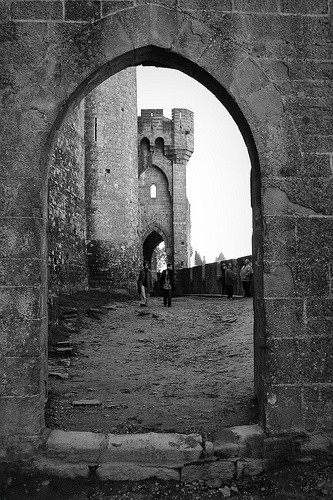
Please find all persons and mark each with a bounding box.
[159,264,174,307]
[137,261,152,307]
[219,263,227,295]
[240,259,253,297]
[225,265,236,300]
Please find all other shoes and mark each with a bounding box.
[140,304,147,307]
